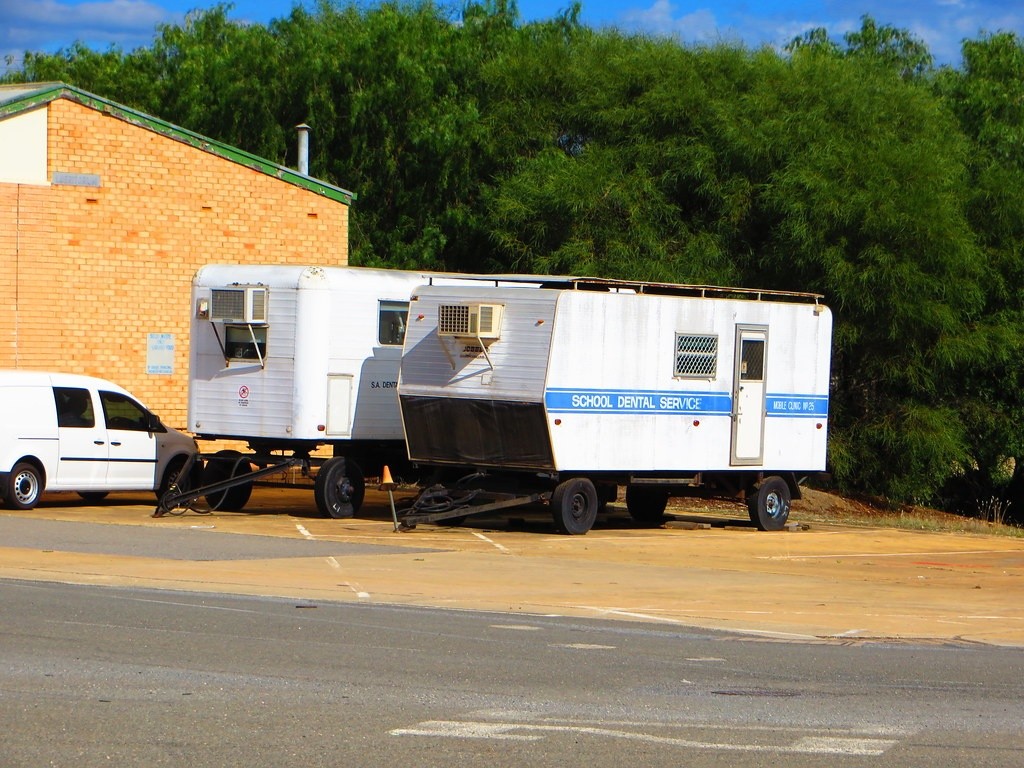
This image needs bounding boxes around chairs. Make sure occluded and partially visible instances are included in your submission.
[63,396,90,426]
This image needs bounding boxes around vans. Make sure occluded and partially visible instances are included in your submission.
[0,370,201,509]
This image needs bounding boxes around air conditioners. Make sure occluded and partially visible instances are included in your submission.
[209,283,269,323]
[437,303,504,341]
